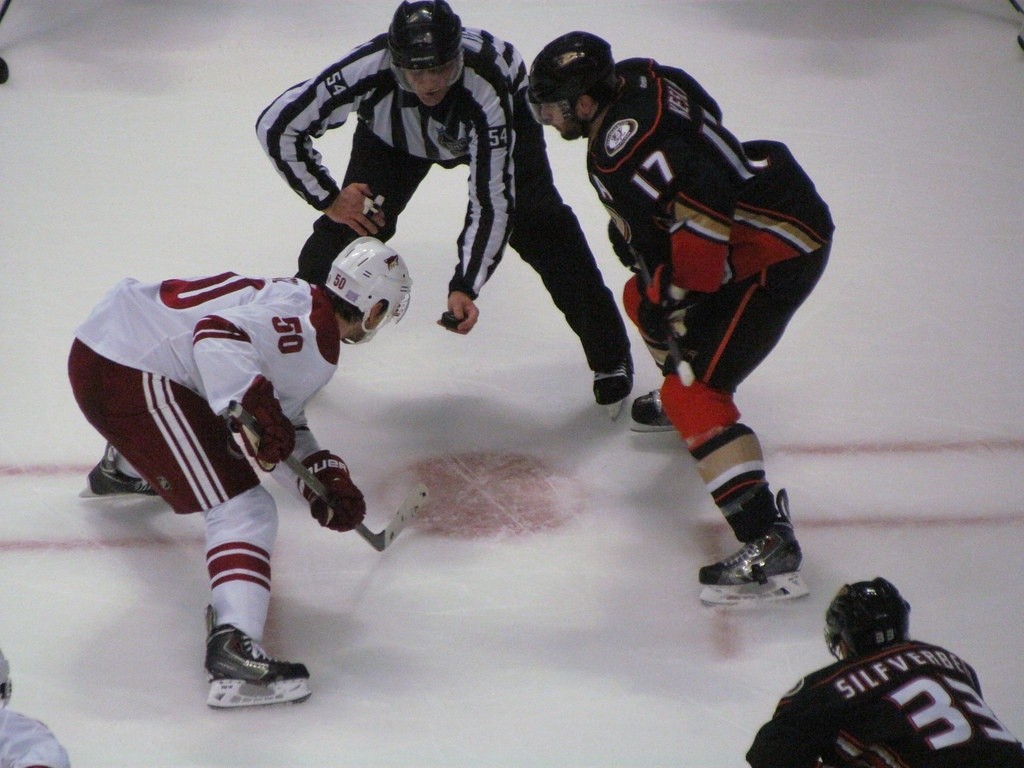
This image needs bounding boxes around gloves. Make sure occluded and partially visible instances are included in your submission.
[638,283,719,345]
[608,216,658,271]
[297,450,367,532]
[228,375,296,463]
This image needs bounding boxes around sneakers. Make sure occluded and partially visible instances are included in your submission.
[79,441,159,501]
[698,488,810,603]
[630,386,679,433]
[205,604,311,708]
[593,351,633,421]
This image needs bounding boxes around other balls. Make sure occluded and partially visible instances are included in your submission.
[441,308,471,328]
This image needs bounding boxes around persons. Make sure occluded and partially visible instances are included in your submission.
[253,0,635,425]
[0,646,70,768]
[747,574,1024,768]
[68,237,413,710]
[523,30,834,605]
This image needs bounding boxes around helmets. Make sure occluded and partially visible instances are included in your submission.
[527,31,616,110]
[325,236,412,331]
[824,577,911,660]
[0,650,12,709]
[388,0,463,72]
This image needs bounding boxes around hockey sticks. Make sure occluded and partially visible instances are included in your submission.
[227,399,430,550]
[619,241,697,388]
